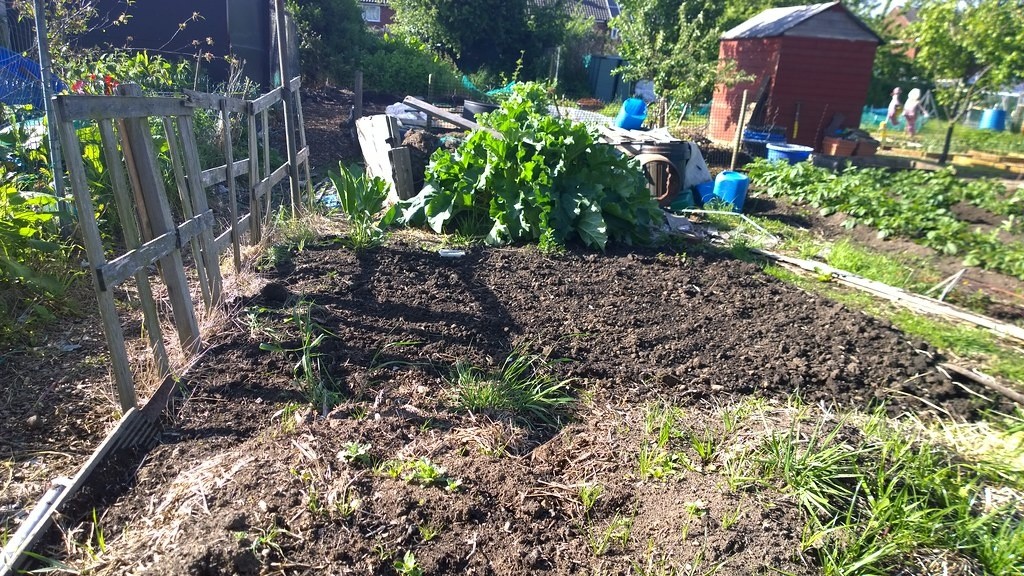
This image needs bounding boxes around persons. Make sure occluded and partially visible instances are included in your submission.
[904,88,928,136]
[886,87,903,125]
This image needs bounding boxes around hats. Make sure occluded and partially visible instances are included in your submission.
[890,87,903,96]
[908,87,921,101]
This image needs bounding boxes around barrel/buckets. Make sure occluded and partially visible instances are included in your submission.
[713,171,749,211]
[766,142,814,166]
[980,109,1006,131]
[618,97,647,130]
[670,190,694,210]
[695,180,715,207]
[460,99,501,133]
